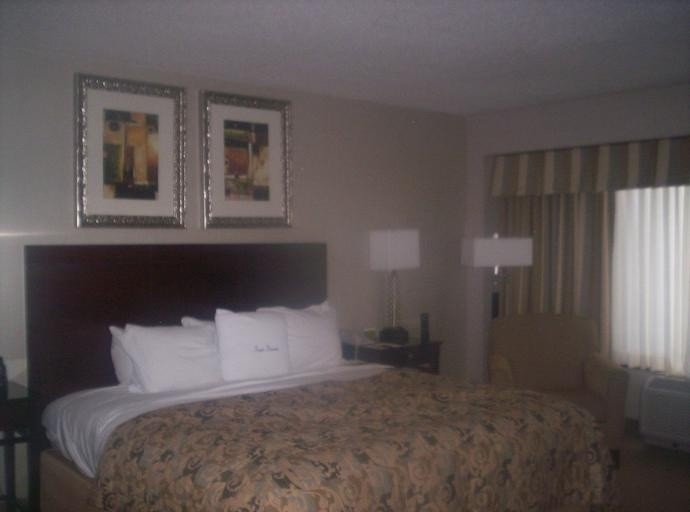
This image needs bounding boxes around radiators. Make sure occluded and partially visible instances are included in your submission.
[636,374,690,454]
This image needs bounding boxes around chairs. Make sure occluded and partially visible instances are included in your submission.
[485,311,631,468]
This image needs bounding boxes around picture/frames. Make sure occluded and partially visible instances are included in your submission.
[198,89,293,227]
[70,72,187,230]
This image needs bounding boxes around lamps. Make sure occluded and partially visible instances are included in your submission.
[368,226,420,342]
[472,230,533,316]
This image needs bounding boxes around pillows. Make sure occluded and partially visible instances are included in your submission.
[106,323,154,395]
[214,308,291,380]
[122,321,223,391]
[261,297,344,373]
[180,316,214,327]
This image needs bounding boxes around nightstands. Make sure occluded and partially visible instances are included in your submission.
[0,359,31,512]
[343,336,444,374]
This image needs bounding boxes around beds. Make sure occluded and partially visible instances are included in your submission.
[23,242,622,512]
